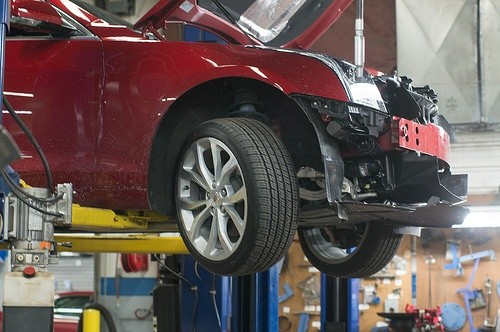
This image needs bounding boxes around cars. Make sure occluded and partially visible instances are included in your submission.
[1,0,469,277]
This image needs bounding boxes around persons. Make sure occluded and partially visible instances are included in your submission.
[372,291,379,304]
